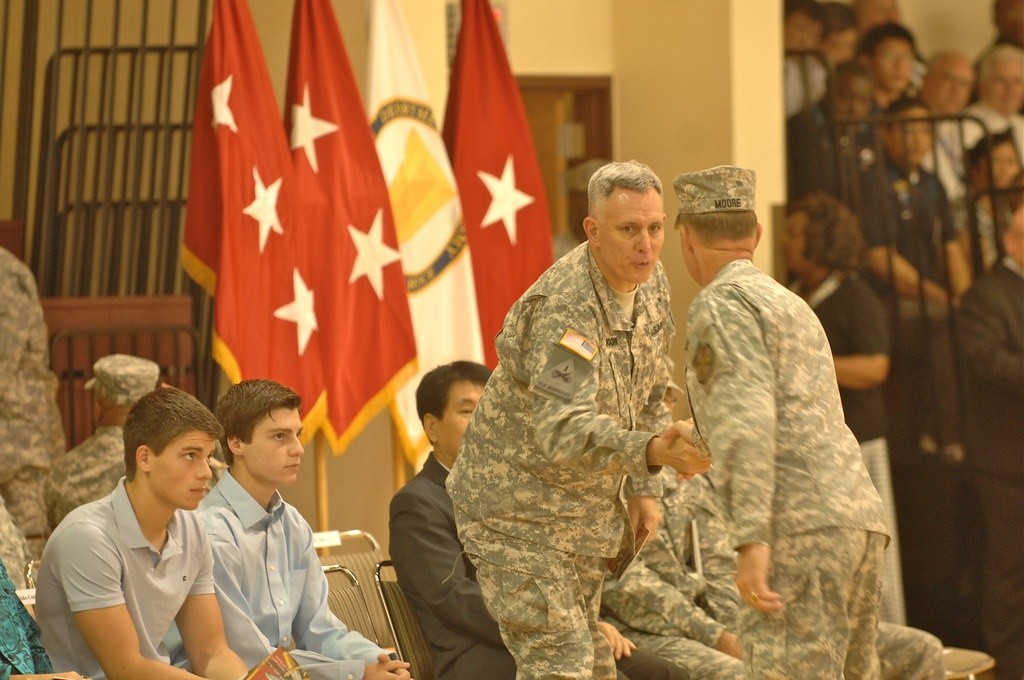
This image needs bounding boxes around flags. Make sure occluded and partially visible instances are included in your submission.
[181,0,553,465]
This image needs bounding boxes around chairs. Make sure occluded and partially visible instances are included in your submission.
[319,565,381,647]
[372,561,436,680]
[311,530,395,649]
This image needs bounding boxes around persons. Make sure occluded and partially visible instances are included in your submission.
[0,558,87,680]
[600,356,947,680]
[0,247,224,593]
[389,360,690,680]
[35,387,248,680]
[785,0,1024,680]
[660,165,893,680]
[446,160,710,680]
[162,380,411,680]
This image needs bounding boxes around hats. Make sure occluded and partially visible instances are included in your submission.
[673,164,761,217]
[85,352,161,408]
